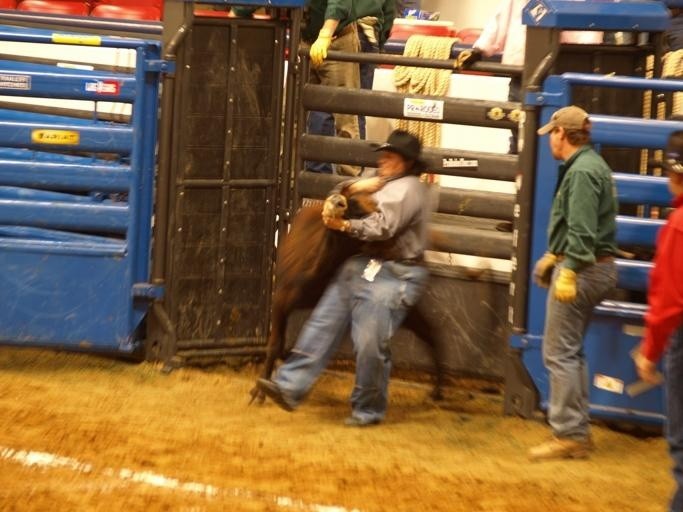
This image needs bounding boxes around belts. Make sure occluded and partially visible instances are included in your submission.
[556,254,613,264]
[332,22,357,41]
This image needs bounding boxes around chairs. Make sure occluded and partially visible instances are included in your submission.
[194,11,272,19]
[382,19,501,77]
[2,1,164,24]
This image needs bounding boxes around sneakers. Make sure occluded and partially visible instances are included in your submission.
[528,432,595,459]
[257,378,293,411]
[497,222,513,232]
[346,416,380,426]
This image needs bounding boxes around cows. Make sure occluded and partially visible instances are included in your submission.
[248,177,451,409]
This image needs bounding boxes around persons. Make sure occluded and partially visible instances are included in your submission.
[529,105,617,460]
[453,0,603,231]
[229,0,362,175]
[632,130,683,512]
[302,0,396,174]
[256,130,428,426]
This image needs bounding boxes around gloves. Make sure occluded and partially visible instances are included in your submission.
[554,266,577,304]
[534,253,556,288]
[454,48,480,70]
[309,30,332,65]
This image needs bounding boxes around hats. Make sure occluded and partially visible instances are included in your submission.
[376,129,420,171]
[537,105,591,135]
[646,131,683,174]
